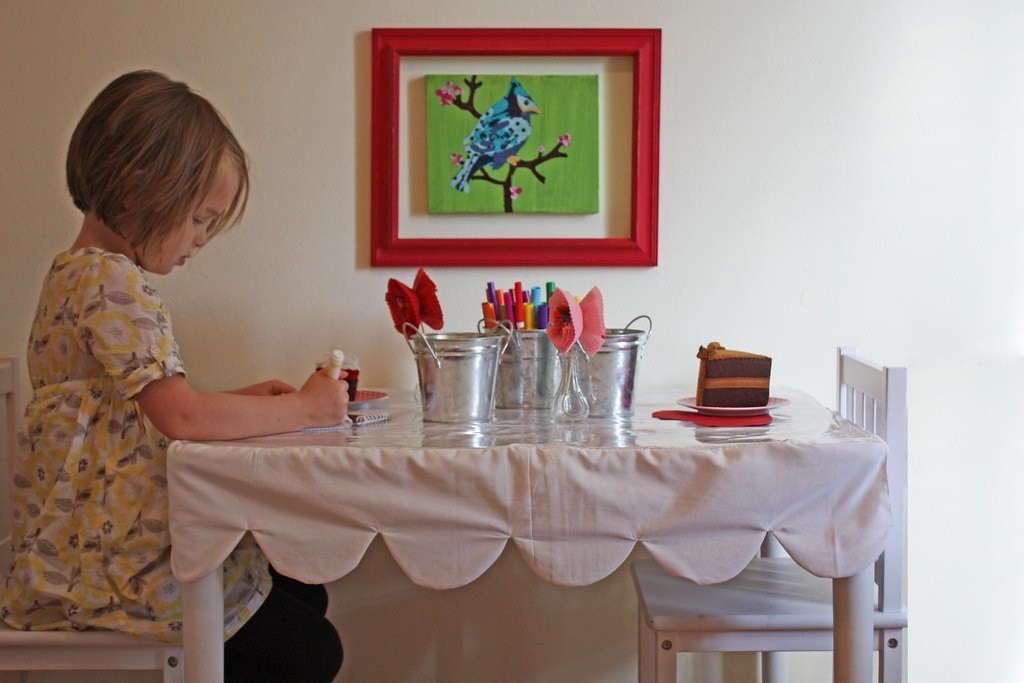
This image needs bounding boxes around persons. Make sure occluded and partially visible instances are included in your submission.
[0,71,350,683]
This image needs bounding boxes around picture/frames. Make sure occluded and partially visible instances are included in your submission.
[369,26,662,269]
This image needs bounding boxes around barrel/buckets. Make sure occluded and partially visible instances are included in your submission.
[483,321,567,410]
[400,320,512,423]
[574,315,652,417]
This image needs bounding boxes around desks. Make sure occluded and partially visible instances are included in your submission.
[166,388,894,683]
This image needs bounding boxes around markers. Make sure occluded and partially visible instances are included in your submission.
[481,281,554,331]
[328,348,345,382]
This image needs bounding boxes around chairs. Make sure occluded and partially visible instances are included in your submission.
[628,345,909,683]
[0,356,185,683]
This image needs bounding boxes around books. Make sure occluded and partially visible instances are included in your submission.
[302,412,390,431]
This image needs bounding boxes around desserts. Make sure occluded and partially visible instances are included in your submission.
[315,352,360,402]
[696,341,772,409]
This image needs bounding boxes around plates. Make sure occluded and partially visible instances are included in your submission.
[675,396,790,415]
[348,389,390,412]
